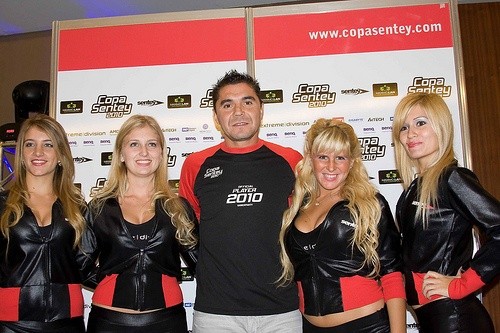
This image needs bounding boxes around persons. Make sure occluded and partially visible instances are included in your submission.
[79,115,200,332]
[179,71,306,332]
[391,90,500,333]
[0,115,94,332]
[281,117,407,333]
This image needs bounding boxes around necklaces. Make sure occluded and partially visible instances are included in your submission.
[315,197,323,206]
